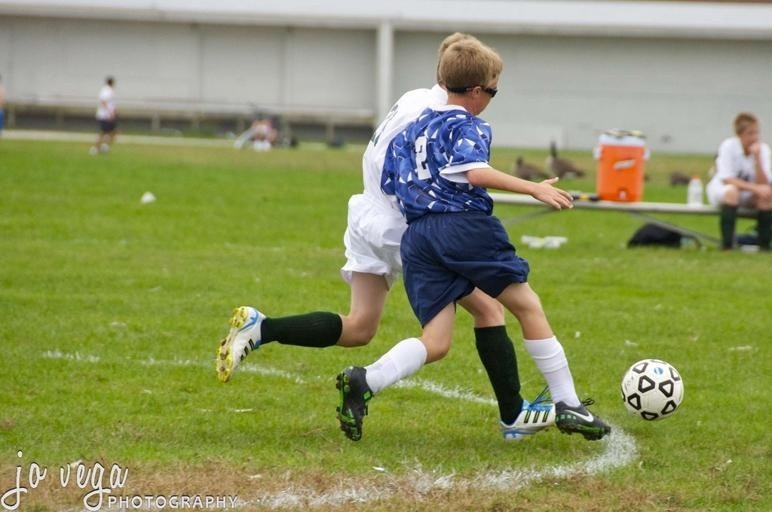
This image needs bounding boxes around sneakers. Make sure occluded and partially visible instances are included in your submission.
[335,366,372,441]
[216,306,267,382]
[556,397,611,440]
[499,387,555,440]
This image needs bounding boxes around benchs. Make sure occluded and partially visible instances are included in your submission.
[487,192,759,251]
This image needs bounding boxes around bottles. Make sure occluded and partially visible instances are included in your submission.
[687,175,704,209]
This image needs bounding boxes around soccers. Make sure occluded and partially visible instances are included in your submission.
[621,359,683,422]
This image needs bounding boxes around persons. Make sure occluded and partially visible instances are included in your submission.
[251,106,273,151]
[545,142,586,180]
[335,37,612,448]
[707,114,771,250]
[95,76,121,155]
[217,31,555,441]
[515,157,551,181]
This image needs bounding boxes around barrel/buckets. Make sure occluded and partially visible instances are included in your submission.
[595,134,651,201]
[686,175,703,204]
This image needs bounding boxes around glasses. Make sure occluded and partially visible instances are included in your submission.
[448,82,498,99]
